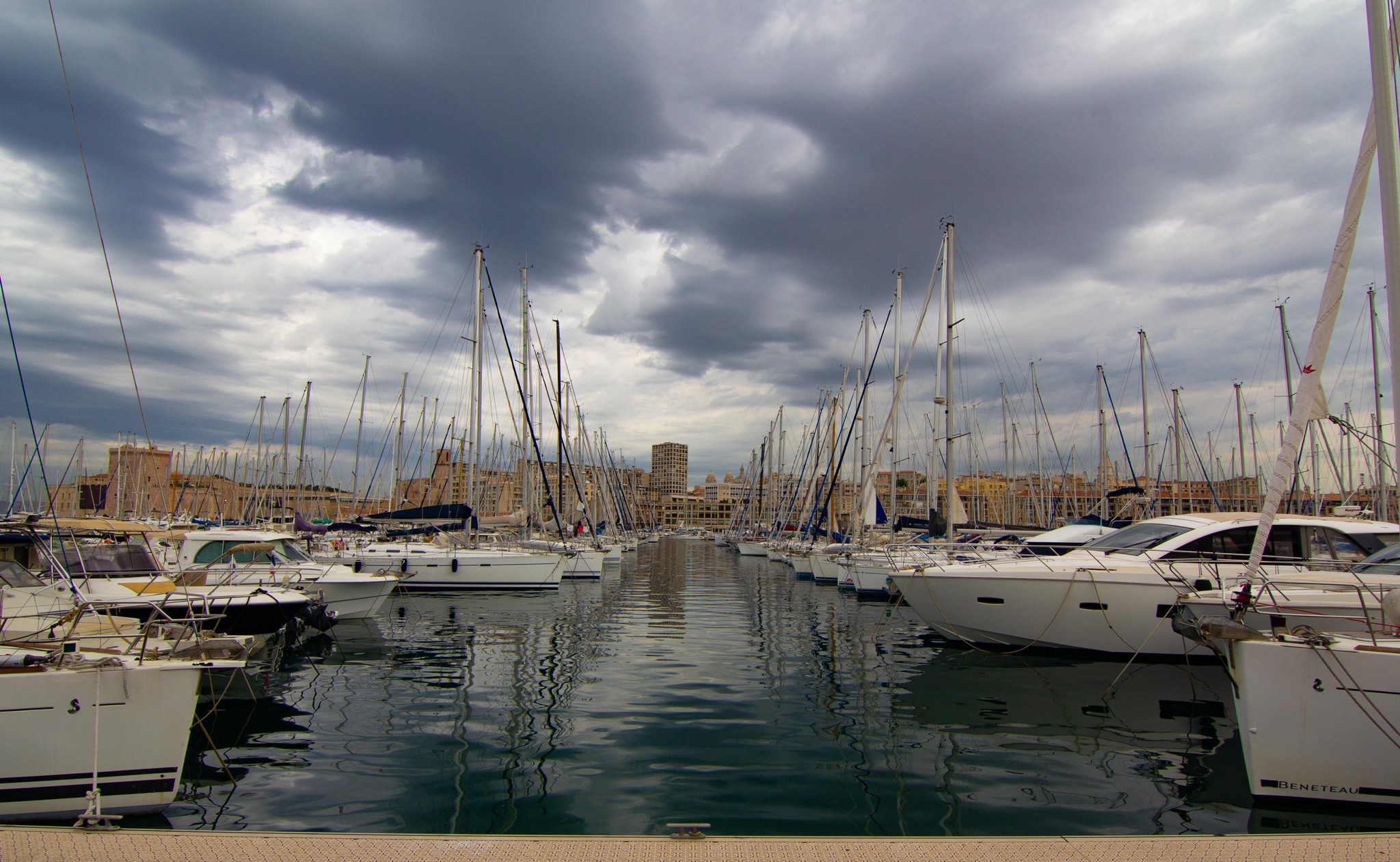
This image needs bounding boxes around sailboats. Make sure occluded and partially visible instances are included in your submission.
[711,0,1399,811]
[0,1,705,824]
